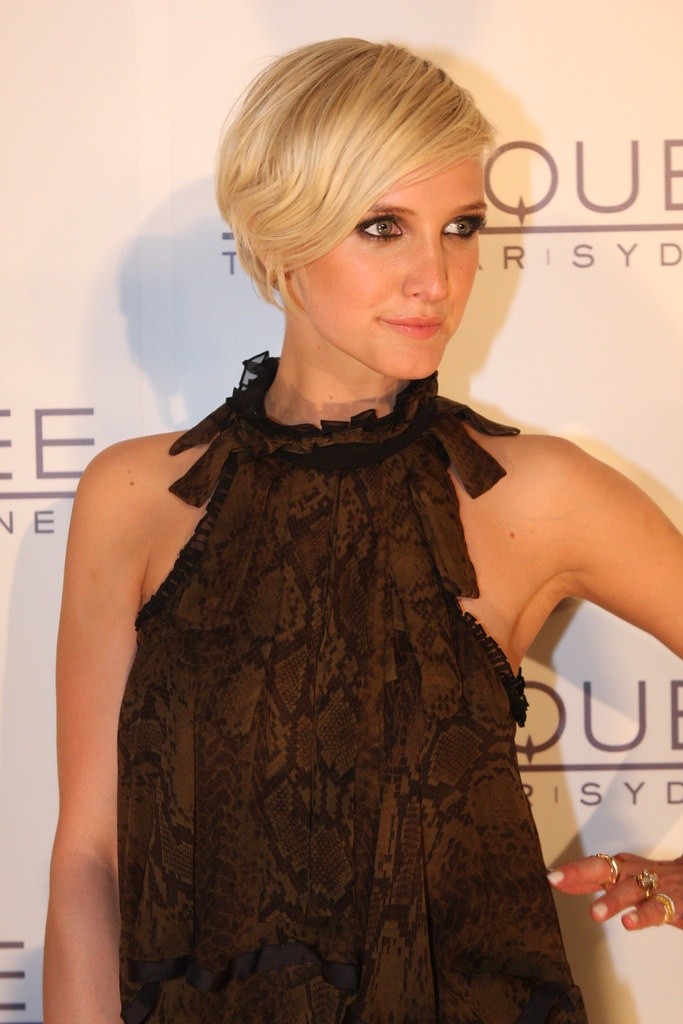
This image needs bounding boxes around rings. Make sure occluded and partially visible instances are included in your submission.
[596,853,618,886]
[655,894,674,926]
[630,869,658,898]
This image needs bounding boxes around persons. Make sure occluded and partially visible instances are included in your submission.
[44,37,683,1024]
